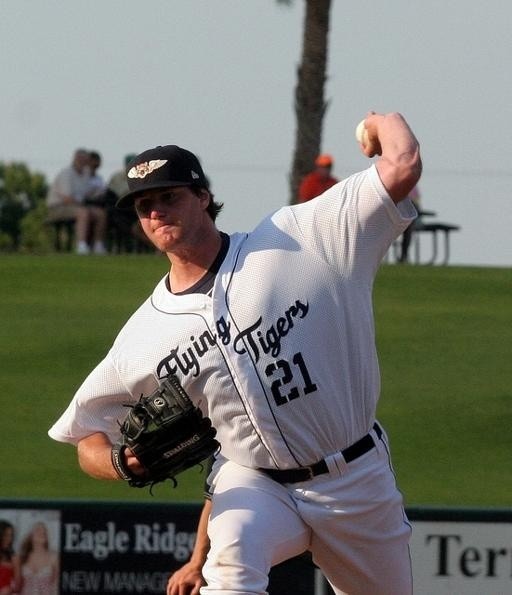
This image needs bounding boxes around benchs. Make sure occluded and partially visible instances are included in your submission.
[385,213,460,265]
[54,216,158,253]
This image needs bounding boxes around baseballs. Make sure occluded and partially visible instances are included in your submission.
[356,119,369,147]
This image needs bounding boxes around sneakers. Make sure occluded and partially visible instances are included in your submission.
[74,240,107,256]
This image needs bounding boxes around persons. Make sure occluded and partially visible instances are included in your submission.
[167,449,227,595]
[298,154,337,204]
[42,149,137,255]
[2,521,20,594]
[47,113,425,595]
[21,520,57,595]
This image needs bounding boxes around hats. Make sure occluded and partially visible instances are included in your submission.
[115,146,209,203]
[315,155,332,168]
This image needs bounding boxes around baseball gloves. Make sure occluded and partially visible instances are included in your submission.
[111,375,220,489]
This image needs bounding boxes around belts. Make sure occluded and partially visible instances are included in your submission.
[258,422,383,484]
